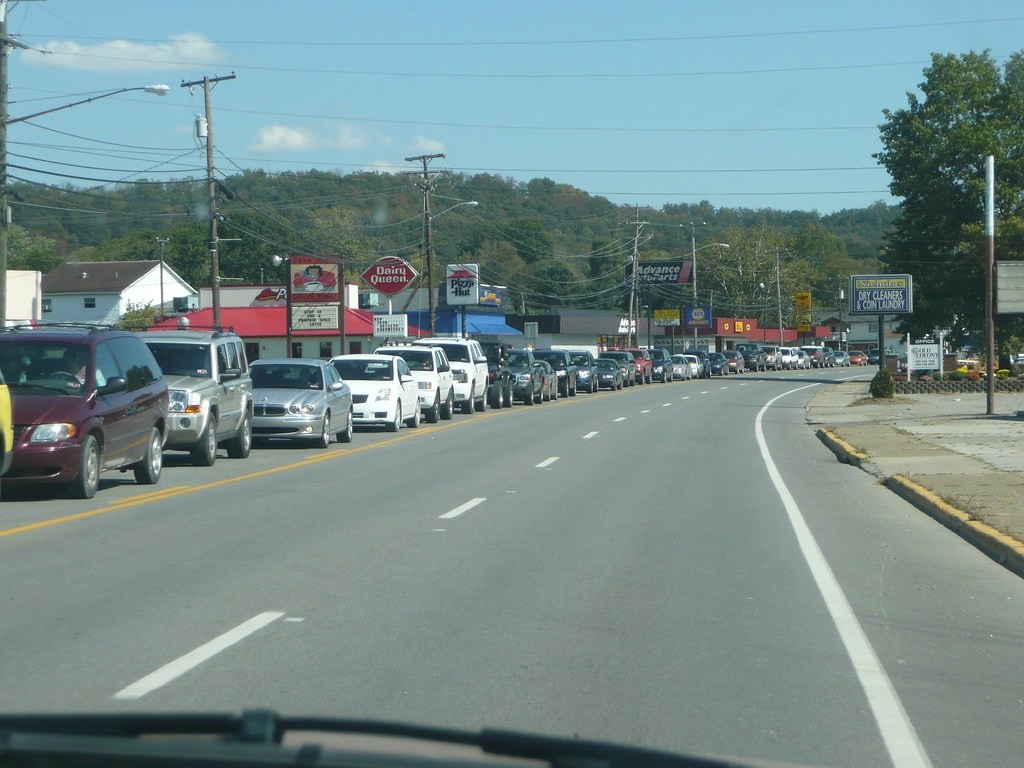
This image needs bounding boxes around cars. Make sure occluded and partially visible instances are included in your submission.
[247,359,354,449]
[507,344,881,406]
[328,354,422,432]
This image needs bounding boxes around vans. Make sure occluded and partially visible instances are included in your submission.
[411,337,492,415]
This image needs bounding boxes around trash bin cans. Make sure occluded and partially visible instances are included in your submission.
[885,354,898,371]
[943,353,958,371]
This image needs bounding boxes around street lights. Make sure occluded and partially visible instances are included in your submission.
[424,200,479,337]
[692,242,731,349]
[0,83,172,319]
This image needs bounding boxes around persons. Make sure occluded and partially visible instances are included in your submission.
[516,355,528,368]
[485,347,496,356]
[41,346,107,388]
[413,353,432,369]
[554,356,564,366]
[605,363,610,369]
[621,357,628,364]
[635,353,642,359]
[655,353,663,361]
[292,369,320,389]
[579,357,587,365]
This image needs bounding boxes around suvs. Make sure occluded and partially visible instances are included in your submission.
[0,321,170,500]
[374,340,456,424]
[470,337,515,410]
[118,324,255,468]
